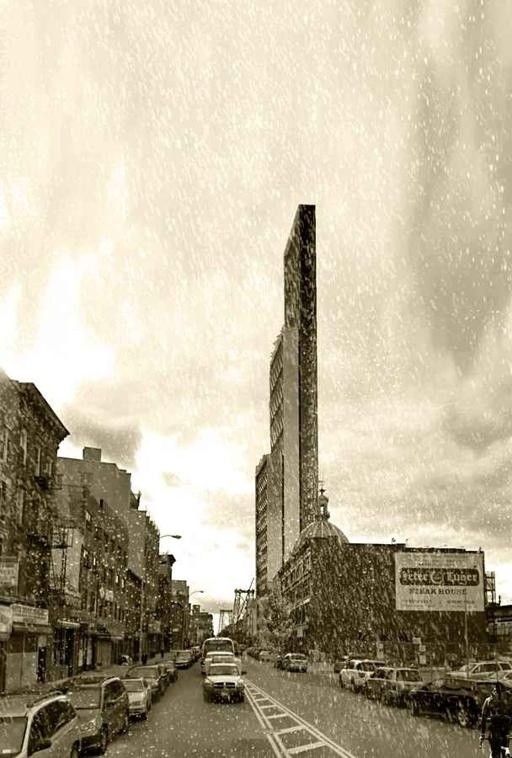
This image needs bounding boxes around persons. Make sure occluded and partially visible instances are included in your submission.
[481,683,512,758]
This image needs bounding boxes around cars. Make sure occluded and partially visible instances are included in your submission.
[173,646,201,668]
[334,656,349,672]
[281,653,308,671]
[247,647,273,660]
[119,661,177,719]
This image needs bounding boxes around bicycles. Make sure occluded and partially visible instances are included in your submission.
[479,737,512,758]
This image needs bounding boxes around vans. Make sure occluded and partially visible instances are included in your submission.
[201,638,247,700]
[404,678,506,728]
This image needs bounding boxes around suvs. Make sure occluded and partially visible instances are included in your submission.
[444,661,512,688]
[57,677,130,754]
[1,690,82,758]
[364,667,423,707]
[338,660,386,693]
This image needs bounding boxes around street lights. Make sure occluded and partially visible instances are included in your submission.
[181,590,204,650]
[139,534,180,664]
